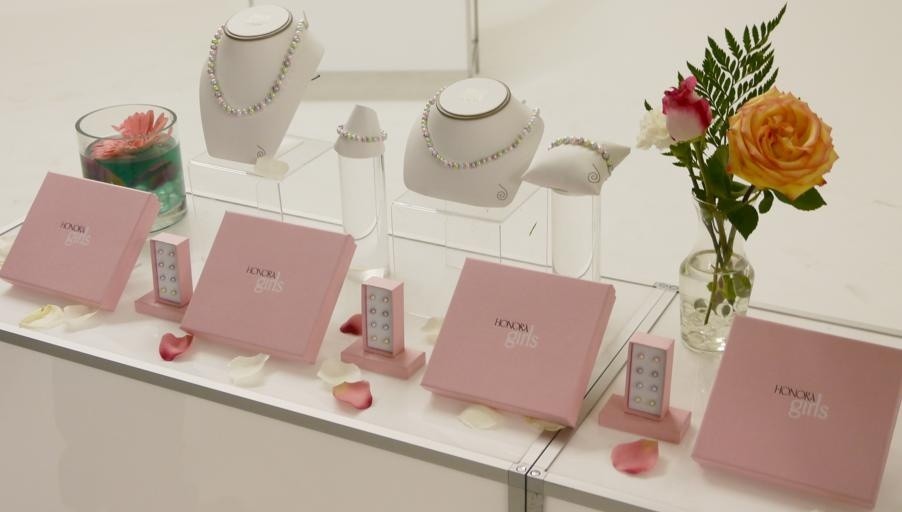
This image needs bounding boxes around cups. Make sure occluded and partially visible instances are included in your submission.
[73,104,189,235]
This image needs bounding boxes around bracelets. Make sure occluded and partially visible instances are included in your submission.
[335,126,388,143]
[546,138,614,173]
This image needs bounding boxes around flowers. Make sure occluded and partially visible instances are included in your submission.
[84,111,176,163]
[643,4,841,318]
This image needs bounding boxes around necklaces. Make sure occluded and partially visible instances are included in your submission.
[421,84,540,168]
[206,19,306,116]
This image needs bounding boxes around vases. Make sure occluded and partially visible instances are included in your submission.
[677,248,756,356]
[74,104,188,235]
